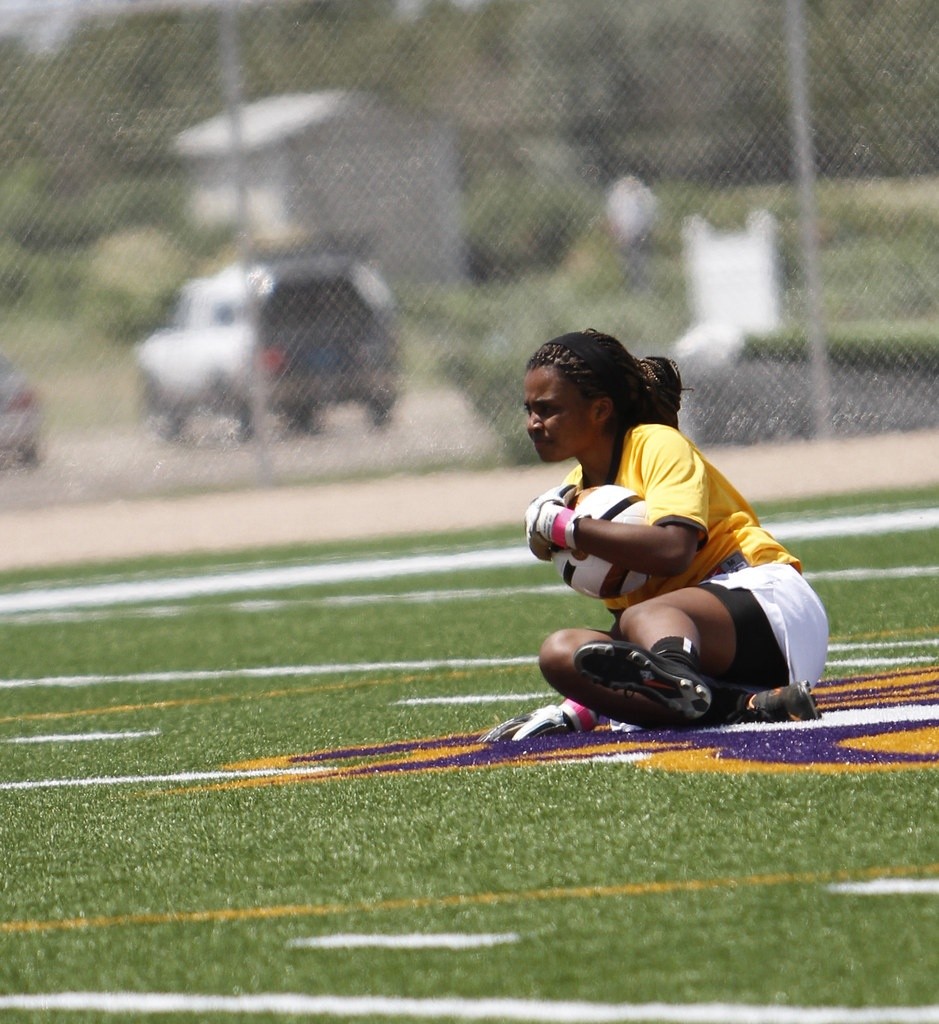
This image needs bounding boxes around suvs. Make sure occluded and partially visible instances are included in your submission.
[135,254,406,445]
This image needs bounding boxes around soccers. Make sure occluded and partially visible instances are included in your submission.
[548,482,655,600]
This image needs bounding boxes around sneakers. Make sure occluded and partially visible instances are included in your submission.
[725,679,823,725]
[575,641,710,719]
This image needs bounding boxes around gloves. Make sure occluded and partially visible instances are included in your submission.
[476,703,584,743]
[524,483,578,562]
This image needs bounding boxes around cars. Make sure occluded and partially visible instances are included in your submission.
[0,346,55,471]
[651,312,825,460]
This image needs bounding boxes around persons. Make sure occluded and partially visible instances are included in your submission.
[476,330,828,743]
